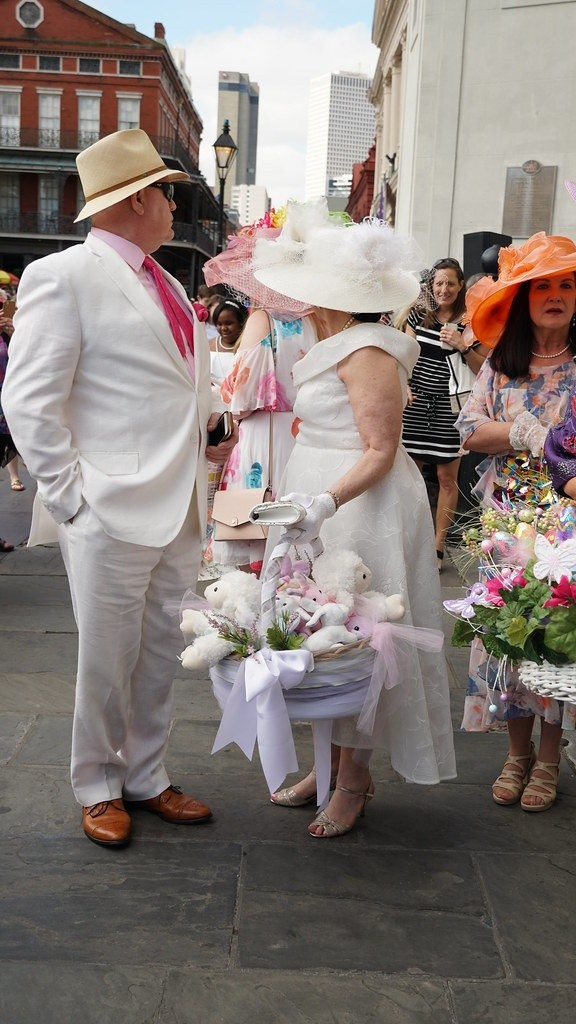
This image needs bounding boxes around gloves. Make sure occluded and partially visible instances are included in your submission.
[280,492,336,543]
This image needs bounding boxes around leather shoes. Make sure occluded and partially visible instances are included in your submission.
[82,798,131,844]
[127,784,212,824]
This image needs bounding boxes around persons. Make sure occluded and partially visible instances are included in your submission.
[0,287,32,491]
[201,218,331,580]
[452,231,576,811]
[0,537,15,552]
[542,390,576,501]
[381,243,501,575]
[0,128,238,848]
[195,283,250,580]
[250,198,456,838]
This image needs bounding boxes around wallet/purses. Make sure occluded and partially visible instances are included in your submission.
[247,501,304,526]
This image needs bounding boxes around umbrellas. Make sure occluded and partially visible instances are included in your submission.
[0,270,20,284]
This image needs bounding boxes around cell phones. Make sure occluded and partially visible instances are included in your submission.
[3,300,15,318]
[210,411,234,447]
[441,322,458,350]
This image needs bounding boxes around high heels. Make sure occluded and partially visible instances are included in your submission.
[491,741,537,803]
[309,775,375,837]
[520,752,561,811]
[270,768,337,806]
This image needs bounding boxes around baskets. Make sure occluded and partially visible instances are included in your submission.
[208,529,389,720]
[517,649,576,703]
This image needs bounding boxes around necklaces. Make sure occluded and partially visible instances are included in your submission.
[531,342,570,358]
[219,335,235,351]
[341,316,356,330]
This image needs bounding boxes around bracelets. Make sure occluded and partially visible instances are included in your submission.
[462,345,471,356]
[325,490,340,511]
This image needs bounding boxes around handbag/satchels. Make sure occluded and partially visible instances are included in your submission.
[212,490,274,541]
[445,352,477,414]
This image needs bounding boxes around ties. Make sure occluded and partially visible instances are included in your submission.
[141,256,194,361]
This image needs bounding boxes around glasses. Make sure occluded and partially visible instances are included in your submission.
[432,258,460,268]
[147,181,174,202]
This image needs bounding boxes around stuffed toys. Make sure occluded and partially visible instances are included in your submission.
[176,547,405,671]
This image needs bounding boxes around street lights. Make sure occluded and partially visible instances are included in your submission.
[212,118,239,298]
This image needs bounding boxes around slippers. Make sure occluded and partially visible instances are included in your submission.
[10,480,24,490]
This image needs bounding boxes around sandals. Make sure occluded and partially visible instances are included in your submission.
[0,537,16,552]
[436,548,444,573]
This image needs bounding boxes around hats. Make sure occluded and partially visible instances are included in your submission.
[73,129,189,223]
[252,195,448,314]
[461,231,576,348]
[481,243,503,276]
[203,206,315,322]
